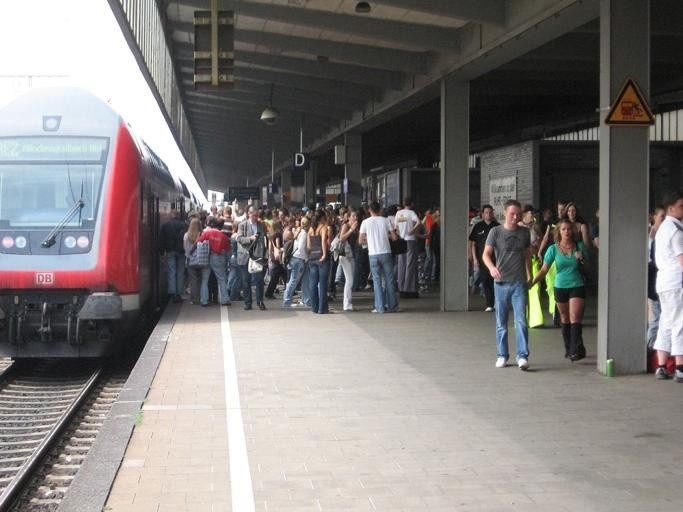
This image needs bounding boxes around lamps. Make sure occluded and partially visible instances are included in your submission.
[255,84,278,126]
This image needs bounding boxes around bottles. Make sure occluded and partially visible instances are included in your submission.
[607,359,615,377]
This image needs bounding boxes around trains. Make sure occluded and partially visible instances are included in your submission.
[0,75,202,371]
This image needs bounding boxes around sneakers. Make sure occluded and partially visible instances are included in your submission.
[171,280,434,313]
[673,368,683,382]
[485,306,494,312]
[518,358,530,370]
[655,366,668,380]
[495,356,507,368]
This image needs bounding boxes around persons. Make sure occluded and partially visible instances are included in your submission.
[481,200,534,369]
[526,218,593,363]
[652,190,683,384]
[646,206,665,362]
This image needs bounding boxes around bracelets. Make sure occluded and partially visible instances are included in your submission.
[579,256,585,265]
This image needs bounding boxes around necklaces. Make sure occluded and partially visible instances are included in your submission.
[559,240,574,249]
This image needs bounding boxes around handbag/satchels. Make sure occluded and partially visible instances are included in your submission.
[247,258,264,274]
[577,243,593,283]
[390,235,408,255]
[329,233,346,259]
[272,240,282,262]
[249,232,268,265]
[188,239,210,268]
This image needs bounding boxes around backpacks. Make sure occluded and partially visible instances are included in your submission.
[282,234,296,268]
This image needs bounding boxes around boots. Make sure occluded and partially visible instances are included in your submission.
[561,323,572,357]
[570,322,586,361]
